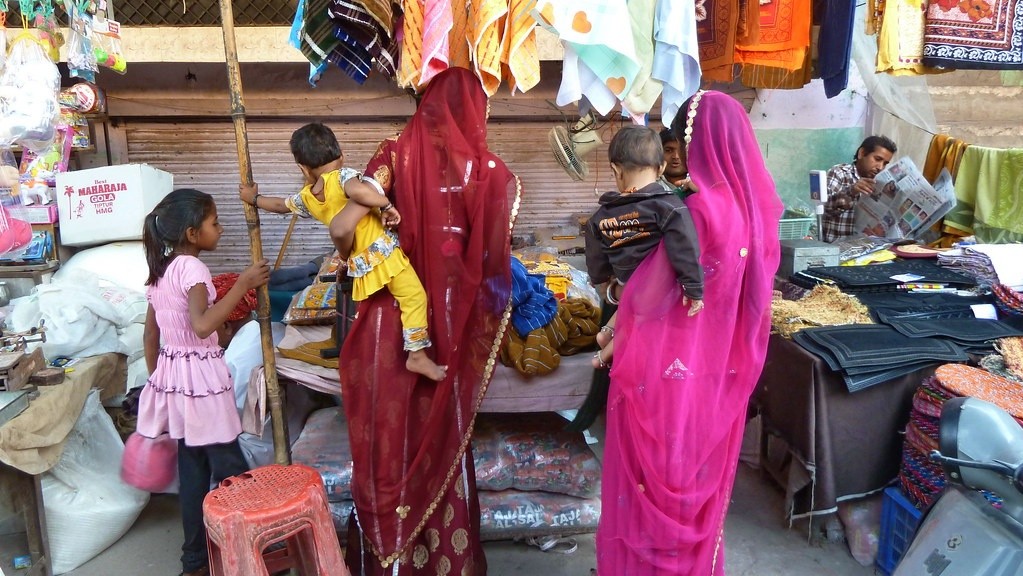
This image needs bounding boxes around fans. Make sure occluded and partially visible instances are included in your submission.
[547,113,603,182]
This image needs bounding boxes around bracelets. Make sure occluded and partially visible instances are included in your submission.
[252,194,260,208]
[380,202,393,212]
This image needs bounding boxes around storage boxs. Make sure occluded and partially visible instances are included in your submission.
[876,487,925,576]
[0,125,174,265]
[776,239,840,280]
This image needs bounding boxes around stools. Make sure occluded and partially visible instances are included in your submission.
[203,464,351,576]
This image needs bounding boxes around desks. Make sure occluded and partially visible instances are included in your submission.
[761,321,987,540]
[0,352,129,576]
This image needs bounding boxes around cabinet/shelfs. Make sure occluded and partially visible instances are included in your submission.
[0,85,112,287]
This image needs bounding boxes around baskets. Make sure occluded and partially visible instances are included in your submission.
[873,486,925,575]
[779,209,817,240]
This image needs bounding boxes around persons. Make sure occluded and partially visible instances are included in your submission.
[581,123,706,362]
[596,87,783,576]
[337,67,524,576]
[238,122,448,383]
[136,188,271,576]
[658,126,688,191]
[822,135,897,246]
